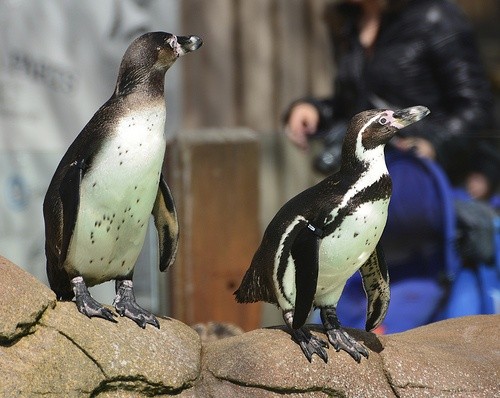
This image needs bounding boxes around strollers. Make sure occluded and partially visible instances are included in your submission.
[310,151,499,336]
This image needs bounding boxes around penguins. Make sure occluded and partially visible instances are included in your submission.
[230,102,432,363]
[41,31,204,331]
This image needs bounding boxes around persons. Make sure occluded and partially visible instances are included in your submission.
[281,0,487,164]
